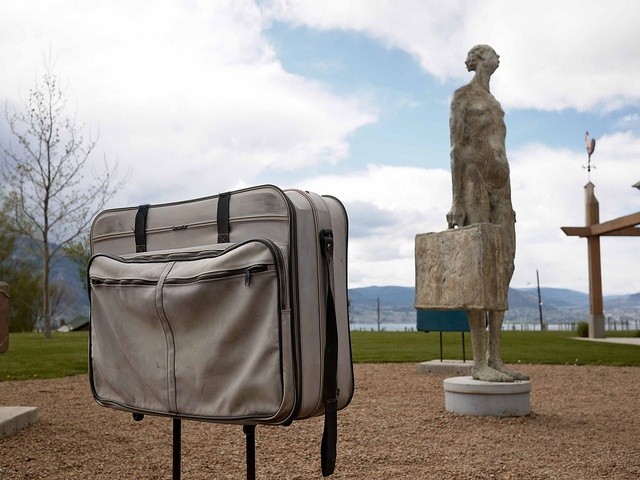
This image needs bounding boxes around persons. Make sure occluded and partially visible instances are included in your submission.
[414,44,532,382]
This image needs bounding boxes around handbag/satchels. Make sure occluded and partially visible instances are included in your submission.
[86,184,356,480]
